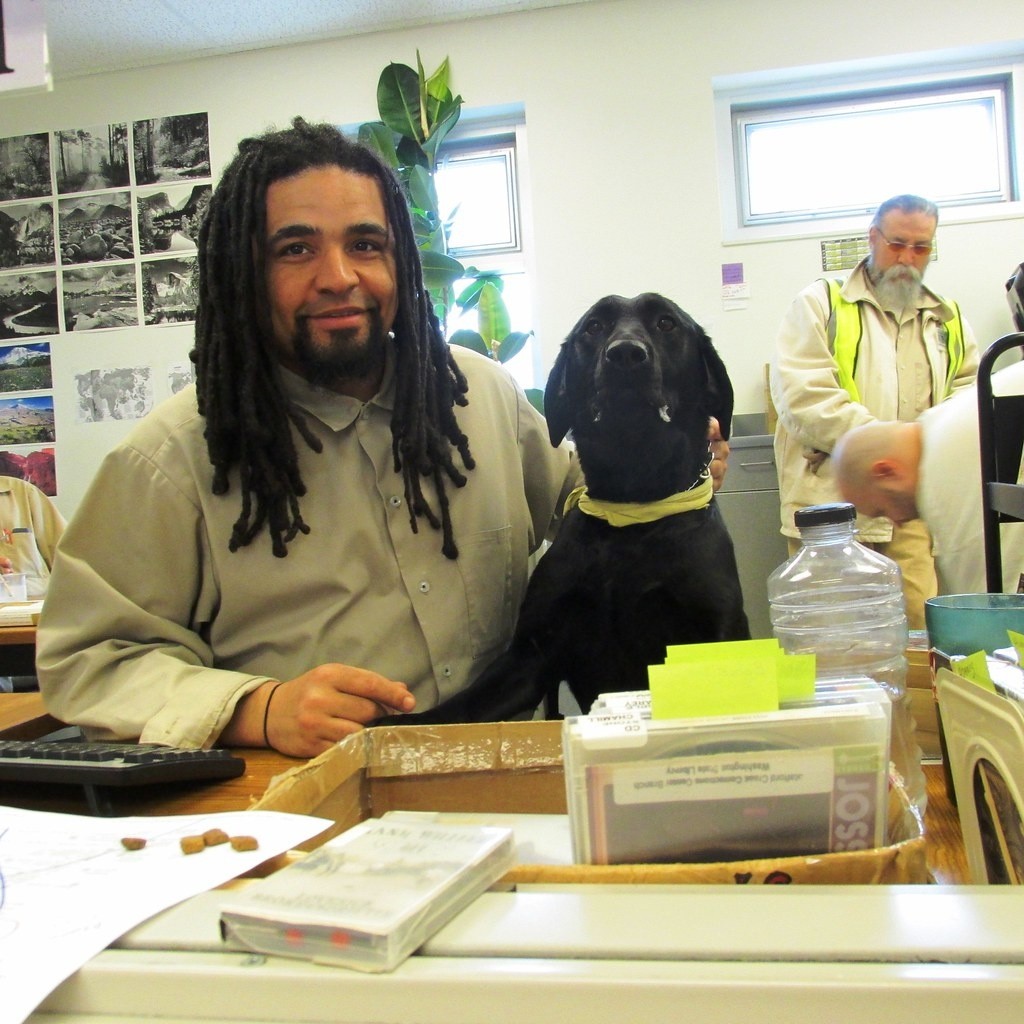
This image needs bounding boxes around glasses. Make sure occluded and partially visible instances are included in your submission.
[877,228,932,257]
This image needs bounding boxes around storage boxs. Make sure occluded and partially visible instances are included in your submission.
[245,724,930,887]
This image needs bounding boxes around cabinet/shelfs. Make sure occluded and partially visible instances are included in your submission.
[714,446,790,640]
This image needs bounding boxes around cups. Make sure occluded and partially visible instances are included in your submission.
[926,593,1022,805]
[0,572,28,602]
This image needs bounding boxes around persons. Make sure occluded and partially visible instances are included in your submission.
[0,476,67,693]
[770,196,981,629]
[35,117,730,757]
[831,360,1024,596]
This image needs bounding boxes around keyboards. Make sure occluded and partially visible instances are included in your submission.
[0,741,246,814]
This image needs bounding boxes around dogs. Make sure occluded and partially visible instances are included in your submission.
[364,290,753,729]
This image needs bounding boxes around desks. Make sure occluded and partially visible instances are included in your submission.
[0,690,972,886]
[0,601,38,646]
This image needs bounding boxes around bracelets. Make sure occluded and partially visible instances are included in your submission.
[263,682,284,748]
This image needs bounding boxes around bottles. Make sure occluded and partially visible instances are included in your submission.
[767,504,907,700]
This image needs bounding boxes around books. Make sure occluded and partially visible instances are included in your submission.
[222,818,518,971]
